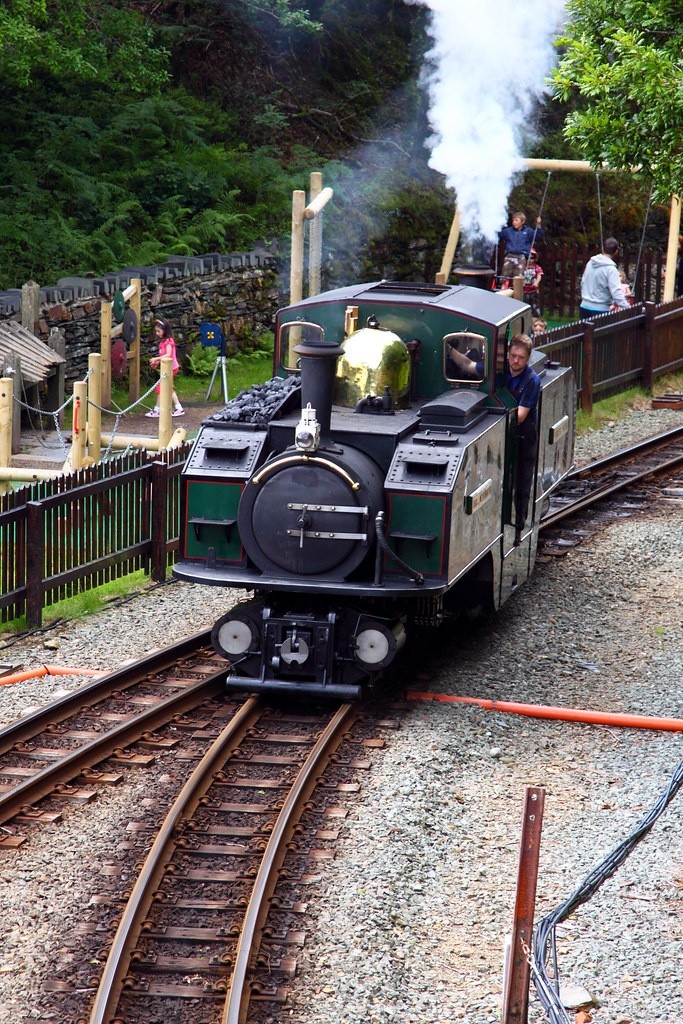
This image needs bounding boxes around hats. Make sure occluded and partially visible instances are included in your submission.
[531,248,537,261]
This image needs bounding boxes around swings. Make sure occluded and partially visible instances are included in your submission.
[491,170,553,292]
[595,171,655,312]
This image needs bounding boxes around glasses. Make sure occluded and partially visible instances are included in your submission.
[530,257,533,259]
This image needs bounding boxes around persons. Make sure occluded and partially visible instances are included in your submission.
[145,318,185,417]
[498,212,548,335]
[580,239,634,319]
[446,336,540,547]
[662,235,683,297]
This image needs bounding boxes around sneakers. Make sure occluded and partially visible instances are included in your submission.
[145,409,159,417]
[170,409,185,417]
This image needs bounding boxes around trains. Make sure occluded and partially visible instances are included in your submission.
[173,265,576,701]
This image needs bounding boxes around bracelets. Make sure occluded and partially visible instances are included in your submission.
[447,347,453,356]
